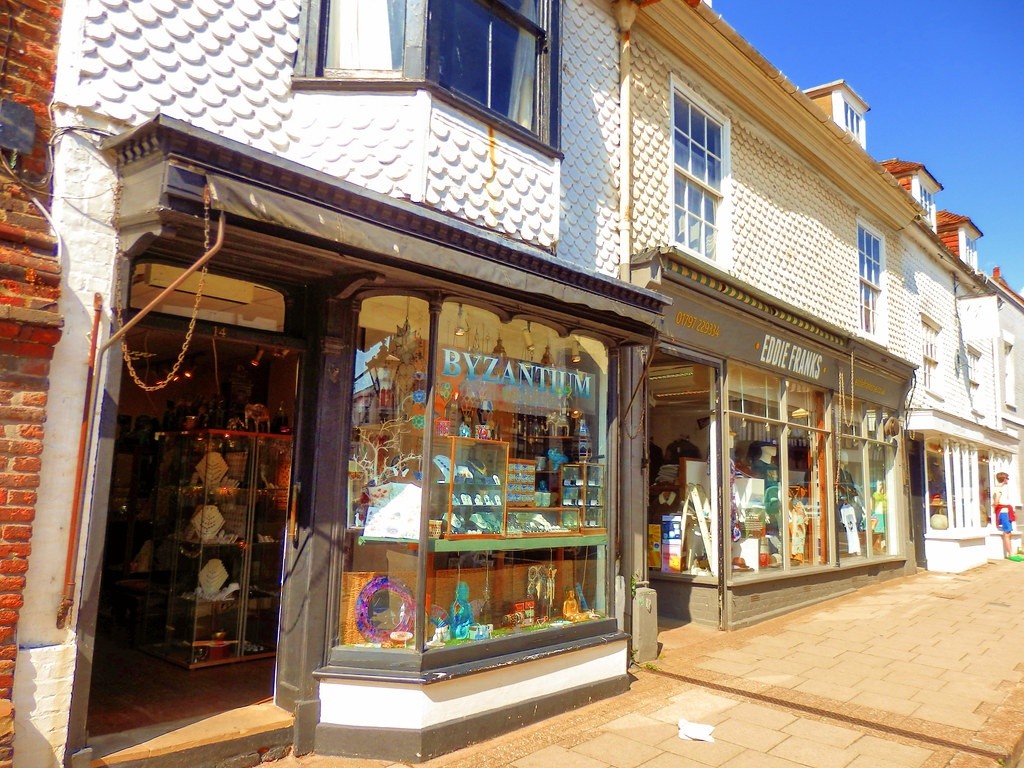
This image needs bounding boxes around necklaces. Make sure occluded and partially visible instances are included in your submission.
[434,455,555,532]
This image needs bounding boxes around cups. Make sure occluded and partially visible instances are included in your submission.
[535,491,551,507]
[428,519,442,539]
[534,456,546,471]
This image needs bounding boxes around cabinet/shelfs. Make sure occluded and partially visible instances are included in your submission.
[428,437,606,541]
[100,431,294,670]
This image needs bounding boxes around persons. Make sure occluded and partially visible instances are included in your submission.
[871,480,885,555]
[991,472,1024,562]
[563,587,589,621]
[751,437,778,553]
[838,449,855,499]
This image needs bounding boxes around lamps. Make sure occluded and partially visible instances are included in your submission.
[151,371,179,384]
[739,369,747,428]
[273,349,290,358]
[455,304,465,336]
[764,374,769,431]
[571,342,581,362]
[185,366,197,377]
[523,320,535,351]
[251,350,265,365]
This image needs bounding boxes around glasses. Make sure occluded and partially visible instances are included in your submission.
[1006,478,1009,480]
